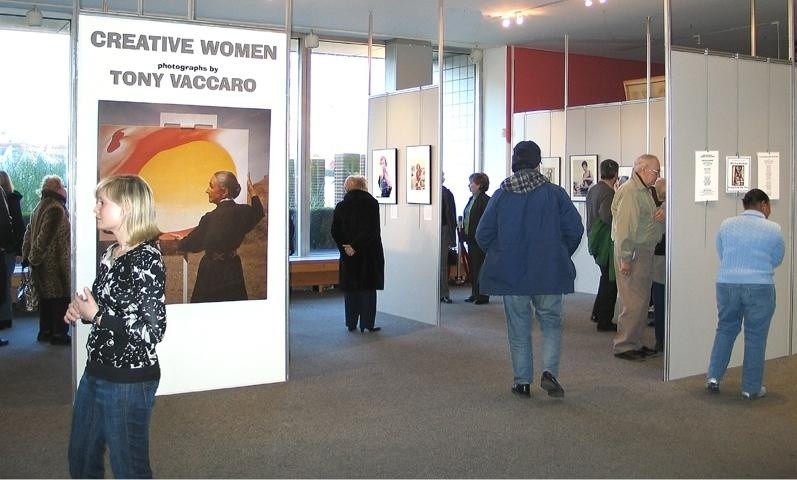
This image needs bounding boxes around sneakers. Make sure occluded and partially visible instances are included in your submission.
[50,334,71,344]
[704,378,720,392]
[740,386,766,401]
[38,331,49,341]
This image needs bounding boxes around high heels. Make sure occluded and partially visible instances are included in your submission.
[360,327,380,332]
[348,325,355,331]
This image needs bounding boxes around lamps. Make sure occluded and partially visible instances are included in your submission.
[26,4,42,26]
[470,44,483,62]
[305,29,319,49]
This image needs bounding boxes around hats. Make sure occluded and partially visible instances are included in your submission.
[512,141,540,171]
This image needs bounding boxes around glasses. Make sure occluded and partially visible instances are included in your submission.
[646,169,660,177]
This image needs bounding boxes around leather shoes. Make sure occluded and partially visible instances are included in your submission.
[473,299,489,303]
[465,296,476,302]
[443,296,452,303]
[598,324,616,331]
[541,371,564,397]
[511,383,530,399]
[614,351,644,362]
[639,347,656,357]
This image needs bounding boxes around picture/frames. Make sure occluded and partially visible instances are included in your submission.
[405,145,431,205]
[371,148,397,205]
[538,157,561,187]
[618,167,633,187]
[568,154,599,202]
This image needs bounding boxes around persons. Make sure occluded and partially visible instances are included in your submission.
[376,157,392,198]
[579,160,595,186]
[169,169,265,303]
[475,141,585,401]
[440,170,458,304]
[413,163,425,191]
[586,153,666,362]
[0,169,25,332]
[699,186,787,400]
[60,174,167,480]
[462,172,492,305]
[329,172,386,334]
[20,174,72,346]
[0,185,11,348]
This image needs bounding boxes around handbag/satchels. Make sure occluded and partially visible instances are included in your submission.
[448,248,457,265]
[16,277,40,313]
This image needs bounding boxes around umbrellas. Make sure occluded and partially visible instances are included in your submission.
[454,227,471,275]
